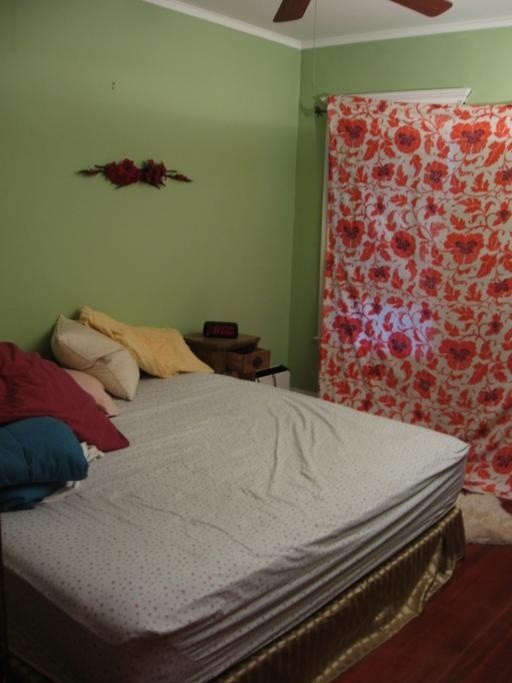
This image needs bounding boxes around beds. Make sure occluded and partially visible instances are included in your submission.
[0,373,469,683]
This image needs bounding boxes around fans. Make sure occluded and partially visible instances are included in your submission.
[273,0,452,22]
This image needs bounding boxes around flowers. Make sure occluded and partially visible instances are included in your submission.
[75,158,195,190]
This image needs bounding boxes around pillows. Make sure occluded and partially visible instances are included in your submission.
[1,339,130,512]
[49,312,142,402]
[78,306,213,377]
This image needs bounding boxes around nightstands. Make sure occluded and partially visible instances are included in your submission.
[184,333,270,375]
